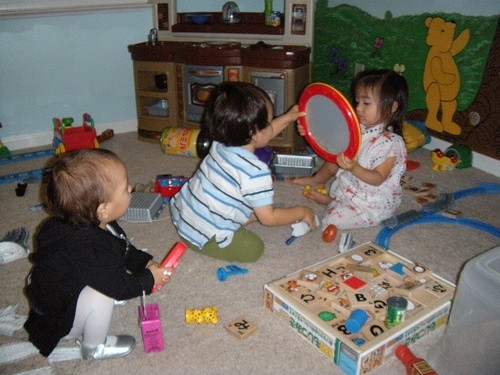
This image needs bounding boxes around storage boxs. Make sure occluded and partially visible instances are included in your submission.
[426,245,500,375]
[263,241,457,375]
[59,126,96,152]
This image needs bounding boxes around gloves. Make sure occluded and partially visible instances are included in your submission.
[0,227,31,265]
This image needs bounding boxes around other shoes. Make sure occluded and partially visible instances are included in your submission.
[80,334,136,360]
[114,299,128,306]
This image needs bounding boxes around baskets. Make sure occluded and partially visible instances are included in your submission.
[119,191,166,222]
[269,153,316,176]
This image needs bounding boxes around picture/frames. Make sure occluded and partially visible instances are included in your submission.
[289,3,306,35]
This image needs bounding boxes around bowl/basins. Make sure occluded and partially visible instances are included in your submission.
[187,14,212,25]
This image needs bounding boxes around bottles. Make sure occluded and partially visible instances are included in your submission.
[160,127,213,160]
[148,28,158,46]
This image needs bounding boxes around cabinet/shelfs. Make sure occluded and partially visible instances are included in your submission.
[128,39,312,153]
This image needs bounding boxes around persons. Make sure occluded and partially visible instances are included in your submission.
[168,81,316,263]
[24,147,176,362]
[286,68,409,232]
